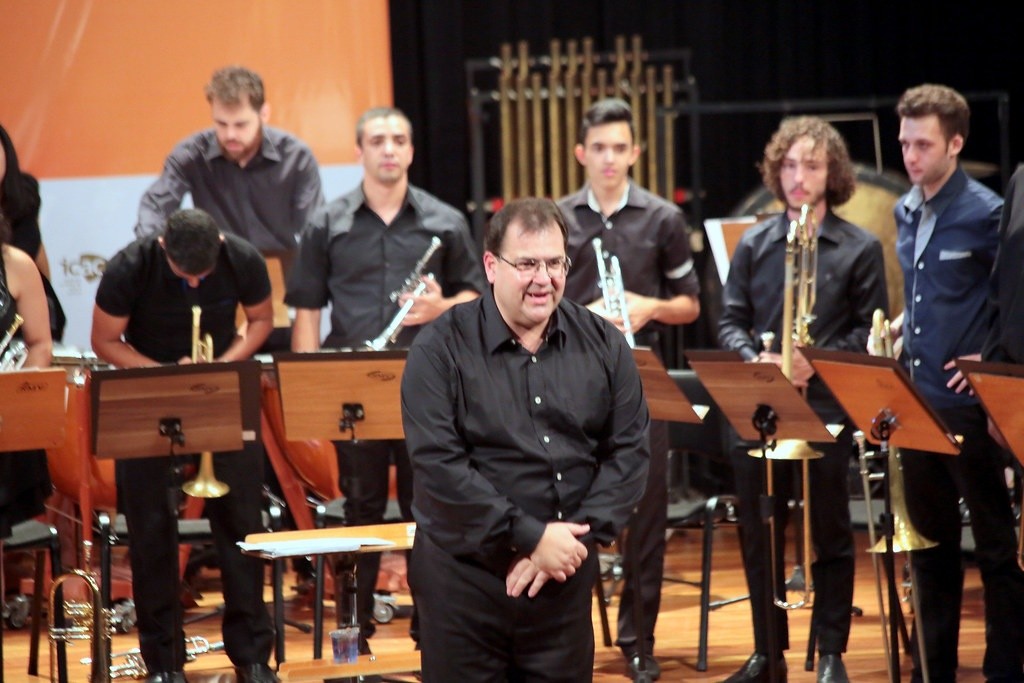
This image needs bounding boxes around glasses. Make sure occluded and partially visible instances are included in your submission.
[494,251,572,276]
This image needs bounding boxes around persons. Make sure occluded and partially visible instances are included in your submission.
[399,197,650,683]
[90,208,282,683]
[283,104,485,656]
[552,100,701,682]
[720,114,888,683]
[136,66,320,335]
[896,83,1024,683]
[0,125,66,541]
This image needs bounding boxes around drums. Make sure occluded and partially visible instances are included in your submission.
[254,348,400,502]
[50,350,201,515]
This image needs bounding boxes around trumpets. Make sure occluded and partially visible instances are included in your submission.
[0,313,30,370]
[46,539,116,683]
[181,304,231,499]
[592,236,636,349]
[363,236,443,349]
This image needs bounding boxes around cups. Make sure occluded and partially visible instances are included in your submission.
[329,628,361,665]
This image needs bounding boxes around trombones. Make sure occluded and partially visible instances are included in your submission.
[746,202,824,611]
[851,308,939,683]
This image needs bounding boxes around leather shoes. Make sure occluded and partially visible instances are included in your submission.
[627,650,661,683]
[816,653,849,683]
[721,649,788,683]
[144,667,190,683]
[237,662,281,683]
